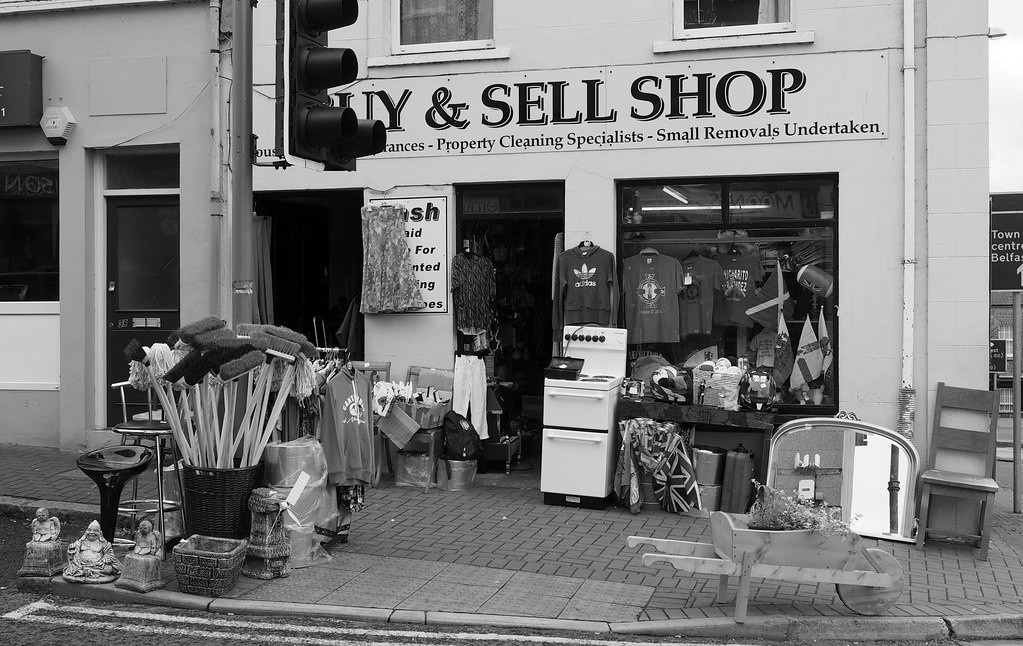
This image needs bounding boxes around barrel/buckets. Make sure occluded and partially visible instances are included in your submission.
[437,459,477,491]
[692,444,729,486]
[699,485,722,511]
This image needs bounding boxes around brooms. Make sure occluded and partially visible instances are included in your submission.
[123,340,183,464]
[160,315,318,475]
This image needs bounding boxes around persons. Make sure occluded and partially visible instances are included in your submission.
[66,520,117,575]
[30,508,57,542]
[135,520,157,555]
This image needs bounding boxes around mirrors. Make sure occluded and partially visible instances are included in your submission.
[766,411,921,544]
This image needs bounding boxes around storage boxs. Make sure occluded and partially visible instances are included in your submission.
[375,399,451,449]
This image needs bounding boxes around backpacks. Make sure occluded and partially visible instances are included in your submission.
[440,411,484,480]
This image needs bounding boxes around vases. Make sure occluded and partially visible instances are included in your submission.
[173,534,248,596]
[747,524,785,532]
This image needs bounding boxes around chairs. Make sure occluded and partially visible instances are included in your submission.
[351,359,457,495]
[916,381,1000,561]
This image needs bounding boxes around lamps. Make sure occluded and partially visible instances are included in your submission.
[661,186,689,204]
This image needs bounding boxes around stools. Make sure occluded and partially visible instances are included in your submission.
[76,444,154,545]
[110,379,186,560]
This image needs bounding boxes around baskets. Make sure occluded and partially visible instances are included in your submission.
[181,458,266,539]
[692,365,747,408]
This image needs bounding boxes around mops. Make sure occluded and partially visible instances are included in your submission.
[255,356,316,466]
[127,338,229,476]
[253,359,289,465]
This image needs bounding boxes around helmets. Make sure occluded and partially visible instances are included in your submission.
[650,364,692,405]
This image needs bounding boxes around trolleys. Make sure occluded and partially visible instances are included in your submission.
[626,510,904,624]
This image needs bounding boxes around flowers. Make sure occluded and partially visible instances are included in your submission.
[744,478,863,545]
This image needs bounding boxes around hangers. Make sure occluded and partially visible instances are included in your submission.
[638,243,659,256]
[577,231,594,248]
[310,346,356,373]
[727,243,742,255]
[680,241,699,261]
[460,240,482,259]
